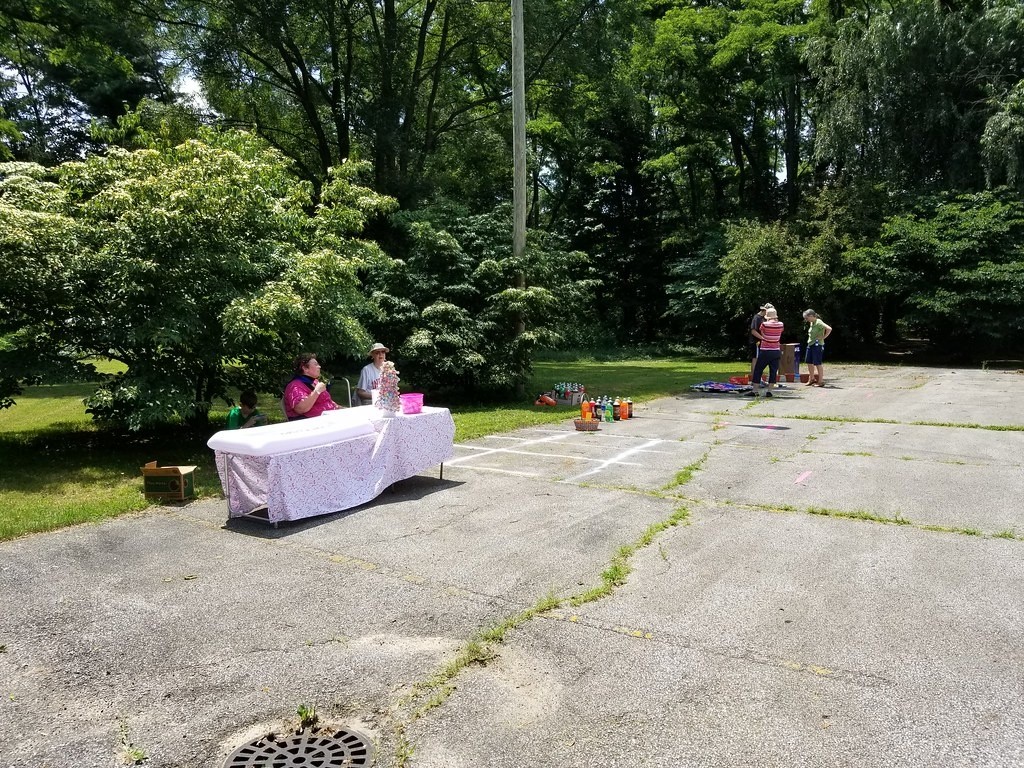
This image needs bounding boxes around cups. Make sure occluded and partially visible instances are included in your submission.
[585,412,592,420]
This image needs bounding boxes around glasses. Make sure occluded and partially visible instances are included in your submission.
[376,350,385,355]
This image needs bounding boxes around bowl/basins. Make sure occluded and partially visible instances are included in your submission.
[762,375,766,381]
[786,375,794,382]
[748,375,753,381]
[776,375,780,382]
[800,374,819,383]
[737,377,749,385]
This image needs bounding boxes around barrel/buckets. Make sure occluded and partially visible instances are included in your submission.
[400,393,424,414]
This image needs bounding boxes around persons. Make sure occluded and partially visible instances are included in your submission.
[284,352,344,421]
[749,303,774,388]
[802,309,833,387]
[227,389,271,430]
[743,308,784,397]
[356,343,389,405]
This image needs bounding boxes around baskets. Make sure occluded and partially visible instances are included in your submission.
[573,393,600,431]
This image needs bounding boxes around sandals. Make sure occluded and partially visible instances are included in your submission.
[805,378,817,386]
[815,381,825,386]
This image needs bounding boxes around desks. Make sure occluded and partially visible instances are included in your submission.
[207,403,456,528]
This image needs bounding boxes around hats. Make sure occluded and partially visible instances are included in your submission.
[764,308,778,319]
[369,343,389,356]
[760,303,774,311]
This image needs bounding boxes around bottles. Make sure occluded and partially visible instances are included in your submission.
[533,382,585,405]
[581,396,632,422]
[815,339,818,346]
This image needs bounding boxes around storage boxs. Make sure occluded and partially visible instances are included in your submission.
[552,390,583,406]
[140,461,197,500]
[777,343,799,375]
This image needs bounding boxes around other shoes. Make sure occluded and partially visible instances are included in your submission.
[751,382,765,388]
[744,391,759,397]
[766,392,773,397]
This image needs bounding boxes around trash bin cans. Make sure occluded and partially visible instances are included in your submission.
[778,343,800,376]
